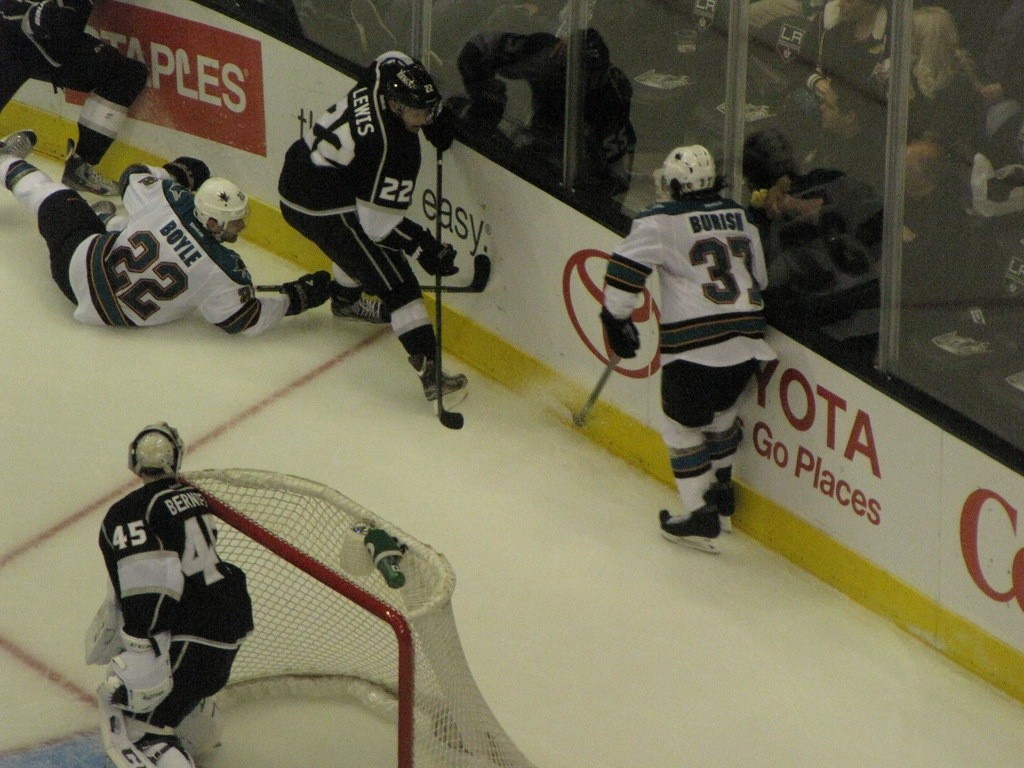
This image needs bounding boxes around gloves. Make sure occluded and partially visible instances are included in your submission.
[420,93,455,151]
[163,157,210,192]
[29,0,79,40]
[405,229,459,276]
[280,271,336,316]
[601,305,640,359]
[476,79,507,129]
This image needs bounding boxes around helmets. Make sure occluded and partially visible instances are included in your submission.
[127,422,184,477]
[743,128,791,182]
[390,64,438,109]
[662,144,715,195]
[193,177,249,235]
[586,27,610,68]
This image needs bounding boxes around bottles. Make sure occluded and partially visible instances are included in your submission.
[556,0,596,38]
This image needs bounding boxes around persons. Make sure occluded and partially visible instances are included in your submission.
[0,0,147,196]
[85,422,254,768]
[224,0,762,238]
[762,0,1024,460]
[460,29,635,238]
[0,129,336,337]
[599,145,777,554]
[740,132,884,380]
[192,1,303,50]
[278,51,469,415]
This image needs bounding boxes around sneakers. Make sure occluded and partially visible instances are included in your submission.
[660,484,720,554]
[409,353,468,413]
[92,201,116,214]
[0,130,37,158]
[332,278,391,324]
[63,138,121,195]
[715,464,735,533]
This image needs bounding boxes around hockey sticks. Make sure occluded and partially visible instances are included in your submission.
[253,255,491,294]
[529,351,620,427]
[435,146,464,430]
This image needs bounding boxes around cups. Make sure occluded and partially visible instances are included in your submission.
[676,30,696,53]
